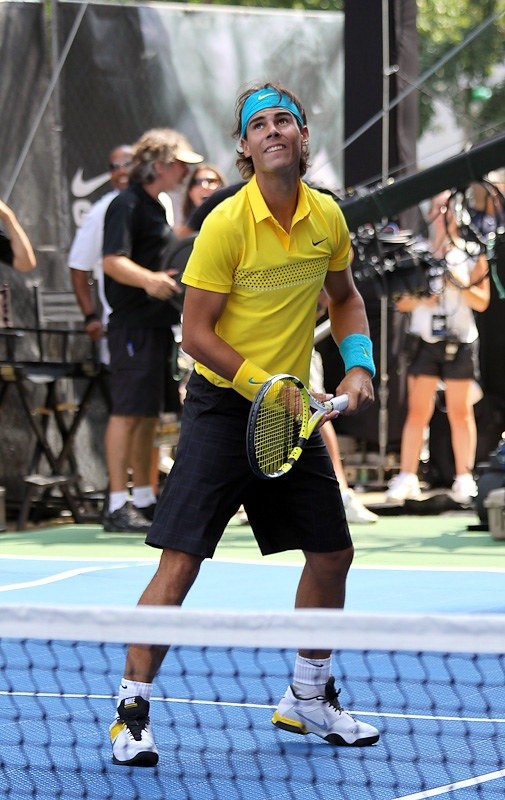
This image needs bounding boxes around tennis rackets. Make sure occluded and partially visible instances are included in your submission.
[244,372,349,482]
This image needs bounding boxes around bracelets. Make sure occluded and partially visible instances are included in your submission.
[338,334,376,379]
[86,314,98,323]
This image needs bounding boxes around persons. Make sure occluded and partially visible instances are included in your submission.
[387,169,505,505]
[66,128,226,534]
[0,197,38,273]
[109,81,380,767]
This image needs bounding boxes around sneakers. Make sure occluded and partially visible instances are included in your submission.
[109,695,160,766]
[272,676,380,746]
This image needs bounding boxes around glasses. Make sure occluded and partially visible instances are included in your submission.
[110,160,138,171]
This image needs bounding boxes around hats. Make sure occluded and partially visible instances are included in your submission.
[142,144,204,164]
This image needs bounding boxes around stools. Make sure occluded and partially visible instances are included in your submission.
[1,326,109,524]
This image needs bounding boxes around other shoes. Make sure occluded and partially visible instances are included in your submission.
[384,472,421,502]
[452,474,477,505]
[104,501,155,533]
